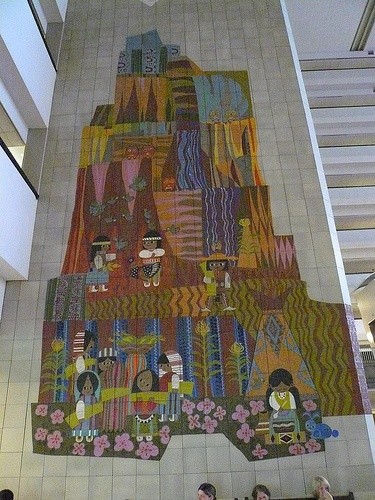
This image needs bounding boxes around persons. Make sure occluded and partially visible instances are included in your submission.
[310,474,335,500]
[0,489,15,500]
[251,483,271,500]
[197,483,218,500]
